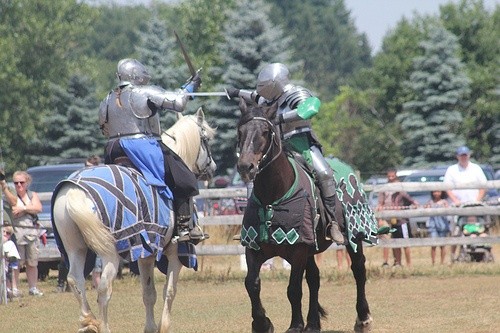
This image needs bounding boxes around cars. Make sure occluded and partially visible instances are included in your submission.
[15,162,85,280]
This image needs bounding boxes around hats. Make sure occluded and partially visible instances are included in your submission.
[457,147,469,155]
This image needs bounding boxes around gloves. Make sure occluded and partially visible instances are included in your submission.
[273,113,284,125]
[227,87,240,98]
[182,67,204,100]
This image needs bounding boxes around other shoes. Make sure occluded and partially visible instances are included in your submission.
[181,230,209,242]
[328,221,345,244]
[29,288,44,296]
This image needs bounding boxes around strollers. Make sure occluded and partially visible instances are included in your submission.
[456,203,496,263]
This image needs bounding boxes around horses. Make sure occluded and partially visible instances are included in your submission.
[52,107,217,333]
[237,96,371,333]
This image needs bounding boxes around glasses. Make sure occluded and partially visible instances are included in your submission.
[14,181,25,184]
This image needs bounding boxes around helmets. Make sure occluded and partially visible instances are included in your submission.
[256,63,290,100]
[116,58,148,86]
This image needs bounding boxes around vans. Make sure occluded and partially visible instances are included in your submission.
[362,162,500,232]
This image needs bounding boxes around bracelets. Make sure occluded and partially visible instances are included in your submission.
[23,206,26,211]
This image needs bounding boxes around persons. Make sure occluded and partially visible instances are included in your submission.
[84,155,103,288]
[462,216,487,251]
[425,191,450,266]
[336,243,350,270]
[378,164,419,267]
[0,169,44,296]
[100,59,209,243]
[443,146,487,266]
[225,62,345,244]
[1,223,21,273]
